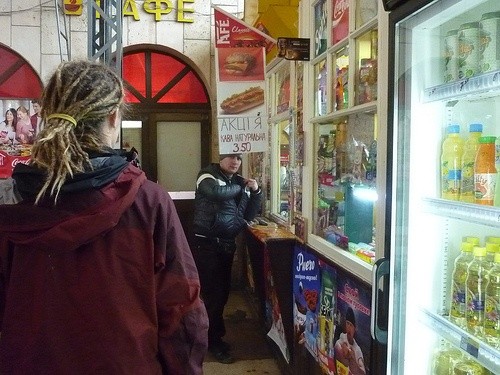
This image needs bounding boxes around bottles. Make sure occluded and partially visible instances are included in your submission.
[441,125,463,200]
[460,124,483,201]
[449,236,500,348]
[493,138,500,207]
[474,137,496,205]
[317,129,337,176]
[432,339,494,375]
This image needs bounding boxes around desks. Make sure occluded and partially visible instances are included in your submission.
[247,224,295,362]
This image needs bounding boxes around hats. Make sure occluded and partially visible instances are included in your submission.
[346,307,356,326]
[218,153,243,162]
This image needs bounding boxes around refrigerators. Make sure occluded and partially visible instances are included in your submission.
[370,0,500,375]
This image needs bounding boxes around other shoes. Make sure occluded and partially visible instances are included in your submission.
[208,340,234,364]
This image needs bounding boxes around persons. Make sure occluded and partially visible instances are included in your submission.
[334,308,367,375]
[0,98,45,144]
[0,59,210,375]
[189,152,264,363]
